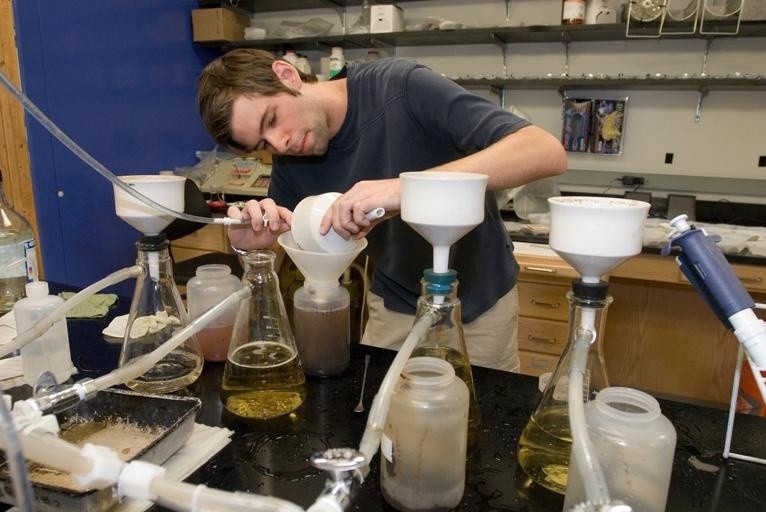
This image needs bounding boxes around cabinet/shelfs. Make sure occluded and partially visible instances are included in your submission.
[516,255,580,376]
[168,211,288,308]
[189,2,766,118]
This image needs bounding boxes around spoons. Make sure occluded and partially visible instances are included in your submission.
[354,353,372,413]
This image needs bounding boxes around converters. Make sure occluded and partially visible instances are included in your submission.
[621,175,644,185]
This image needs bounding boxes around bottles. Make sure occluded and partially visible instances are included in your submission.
[118,237,205,395]
[563,385,677,512]
[0,169,41,317]
[328,45,345,80]
[561,1,586,25]
[184,250,352,419]
[367,51,378,61]
[378,357,470,511]
[281,49,311,75]
[14,283,73,389]
[411,269,487,437]
[519,295,617,498]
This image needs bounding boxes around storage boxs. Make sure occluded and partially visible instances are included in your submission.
[190,9,252,43]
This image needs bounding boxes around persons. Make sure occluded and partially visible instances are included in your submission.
[193,47,569,380]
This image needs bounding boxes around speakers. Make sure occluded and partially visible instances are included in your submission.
[371,3,403,33]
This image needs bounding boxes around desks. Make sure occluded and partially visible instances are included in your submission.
[603,251,766,420]
[1,279,765,512]
